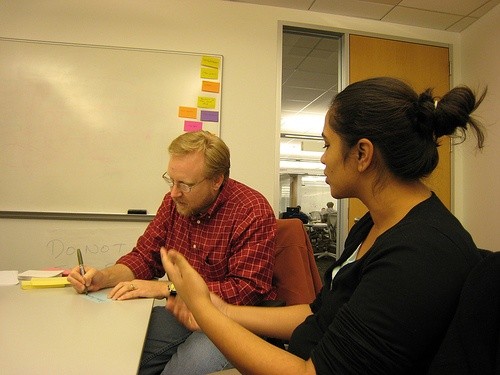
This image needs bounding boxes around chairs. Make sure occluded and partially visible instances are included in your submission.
[435,248,500,375]
[313,214,337,262]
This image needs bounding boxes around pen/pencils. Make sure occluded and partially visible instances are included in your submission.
[77,249,88,295]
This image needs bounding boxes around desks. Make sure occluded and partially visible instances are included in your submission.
[303,223,327,228]
[0,278,159,375]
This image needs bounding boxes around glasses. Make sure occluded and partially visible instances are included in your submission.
[162,172,214,192]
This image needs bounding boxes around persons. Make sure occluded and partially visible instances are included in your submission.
[67,130,283,375]
[322,202,336,215]
[160,77,500,375]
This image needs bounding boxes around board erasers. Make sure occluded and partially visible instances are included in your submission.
[128,209,147,214]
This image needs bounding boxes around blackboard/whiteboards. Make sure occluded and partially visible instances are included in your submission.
[0,36,224,222]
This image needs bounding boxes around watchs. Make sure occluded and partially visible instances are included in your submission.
[167,281,177,299]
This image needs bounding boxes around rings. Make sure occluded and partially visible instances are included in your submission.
[130,284,135,290]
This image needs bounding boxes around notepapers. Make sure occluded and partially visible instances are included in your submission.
[21,277,71,289]
[19,270,63,280]
[44,268,72,276]
